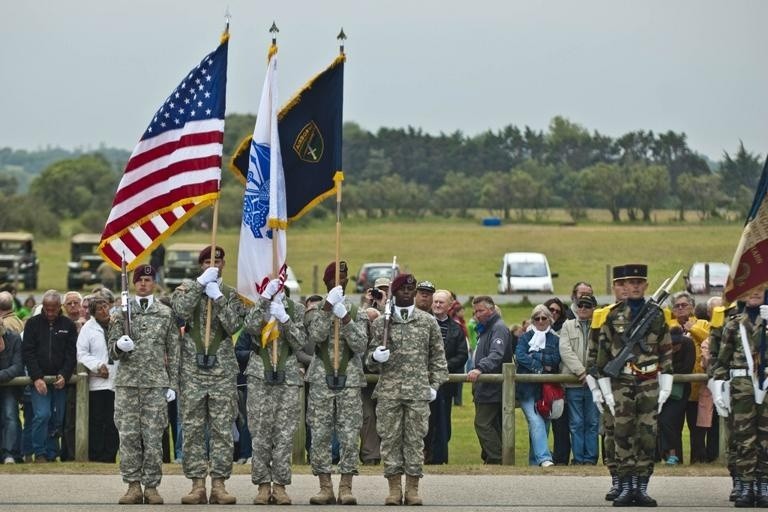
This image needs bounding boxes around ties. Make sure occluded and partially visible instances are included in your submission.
[400,309,408,321]
[139,299,148,309]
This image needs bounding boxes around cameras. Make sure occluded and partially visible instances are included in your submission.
[370,290,381,299]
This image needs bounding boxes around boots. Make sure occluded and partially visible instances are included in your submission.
[310,473,337,504]
[633,477,657,507]
[181,478,208,504]
[630,476,638,506]
[272,482,292,505]
[209,479,236,504]
[734,480,756,507]
[606,475,622,501]
[337,473,358,505]
[757,480,768,508]
[119,481,143,505]
[728,476,742,500]
[404,474,423,506]
[752,480,758,504]
[253,483,272,505]
[614,480,633,507]
[143,484,164,504]
[384,474,403,506]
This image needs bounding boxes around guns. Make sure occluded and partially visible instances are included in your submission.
[378,254,398,370]
[601,267,685,379]
[120,250,133,355]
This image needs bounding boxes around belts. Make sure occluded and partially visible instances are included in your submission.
[621,362,659,375]
[729,368,768,377]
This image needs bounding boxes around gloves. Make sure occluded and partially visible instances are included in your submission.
[270,299,290,324]
[196,267,218,287]
[585,374,605,414]
[206,282,224,303]
[428,387,437,404]
[759,304,768,321]
[597,377,616,416]
[372,346,390,364]
[333,303,348,319]
[165,389,177,402]
[260,279,280,300]
[326,285,343,306]
[657,371,673,415]
[116,334,134,353]
[706,378,729,417]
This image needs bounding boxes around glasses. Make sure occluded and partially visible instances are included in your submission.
[672,303,692,308]
[577,304,593,309]
[65,301,79,305]
[549,308,561,314]
[534,317,547,321]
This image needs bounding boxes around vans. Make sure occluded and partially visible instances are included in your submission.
[495,251,558,294]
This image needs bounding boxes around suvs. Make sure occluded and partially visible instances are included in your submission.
[0,231,37,291]
[68,233,122,290]
[163,242,210,291]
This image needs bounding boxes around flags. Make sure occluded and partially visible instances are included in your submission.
[722,155,768,306]
[98,32,228,271]
[229,53,346,221]
[237,46,288,349]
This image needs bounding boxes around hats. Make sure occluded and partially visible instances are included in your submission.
[623,264,648,279]
[323,260,348,281]
[132,265,155,284]
[391,273,416,296]
[579,295,597,306]
[416,281,435,293]
[374,277,391,288]
[612,266,624,281]
[198,245,225,264]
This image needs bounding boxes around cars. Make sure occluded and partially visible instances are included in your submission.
[350,262,402,294]
[681,260,731,293]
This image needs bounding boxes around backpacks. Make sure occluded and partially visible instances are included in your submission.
[535,374,566,420]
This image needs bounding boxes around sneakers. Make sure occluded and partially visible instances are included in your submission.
[667,455,679,466]
[35,457,48,463]
[4,457,16,465]
[540,461,555,468]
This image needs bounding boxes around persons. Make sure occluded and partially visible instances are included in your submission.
[297,262,369,504]
[587,264,674,506]
[365,274,448,505]
[0,280,768,466]
[240,271,307,504]
[170,245,247,504]
[707,277,768,508]
[106,262,181,506]
